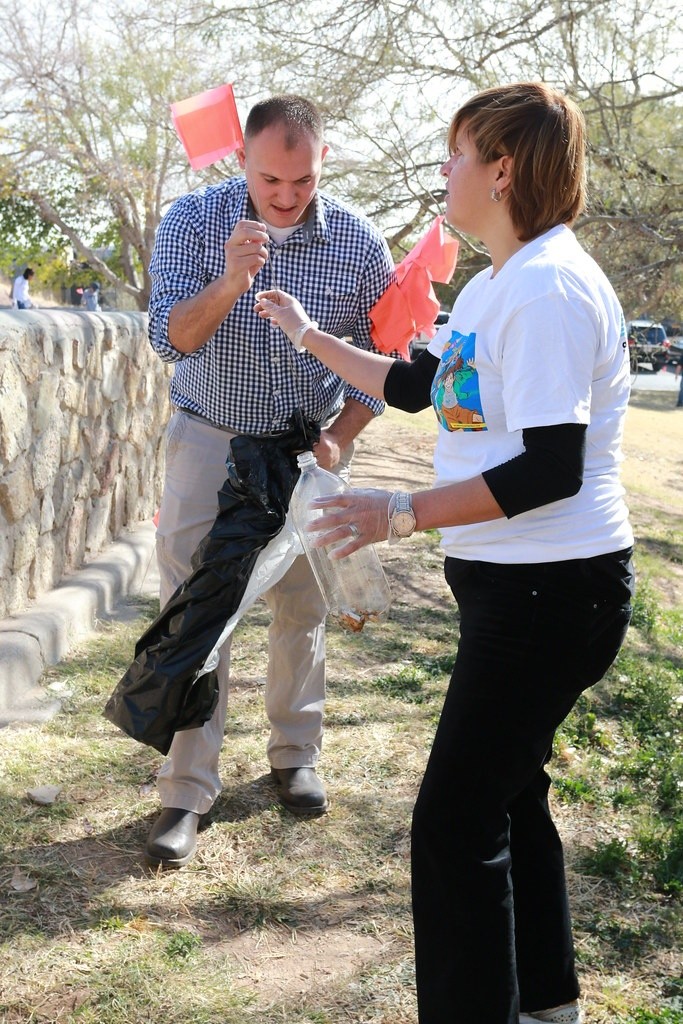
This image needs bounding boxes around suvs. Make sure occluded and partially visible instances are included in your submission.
[409,310,450,359]
[627,320,670,374]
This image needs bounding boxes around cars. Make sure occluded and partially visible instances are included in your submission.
[663,322,683,366]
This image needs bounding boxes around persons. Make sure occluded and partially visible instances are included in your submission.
[145,94,416,868]
[81,282,100,312]
[12,267,35,310]
[674,353,683,408]
[250,83,637,1024]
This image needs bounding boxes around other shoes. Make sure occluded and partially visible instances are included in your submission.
[145,807,199,866]
[520,1015,581,1024]
[271,765,327,813]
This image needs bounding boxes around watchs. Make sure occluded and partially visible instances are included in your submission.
[389,491,418,540]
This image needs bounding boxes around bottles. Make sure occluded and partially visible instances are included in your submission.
[292,453,388,615]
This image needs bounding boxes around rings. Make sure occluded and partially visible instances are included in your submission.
[348,523,359,538]
